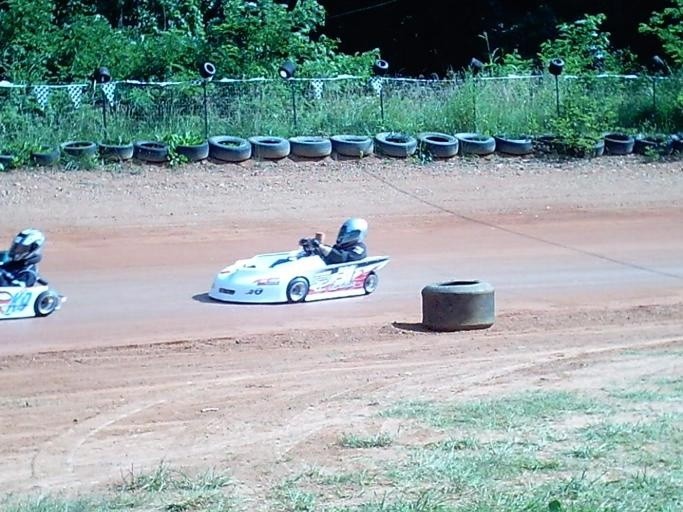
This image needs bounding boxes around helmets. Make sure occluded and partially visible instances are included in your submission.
[10,228,45,266]
[335,217,368,248]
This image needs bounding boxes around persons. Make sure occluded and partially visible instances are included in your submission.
[297,217,370,264]
[0,229,45,288]
